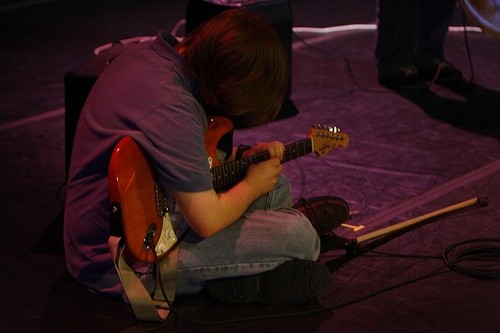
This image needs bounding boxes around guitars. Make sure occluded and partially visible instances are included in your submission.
[107,113,352,265]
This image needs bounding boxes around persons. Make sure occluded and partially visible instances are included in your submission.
[60,10,349,308]
[373,0,466,90]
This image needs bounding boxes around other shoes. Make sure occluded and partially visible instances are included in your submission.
[292,196,349,234]
[207,260,330,304]
[379,72,437,90]
[428,57,462,82]
